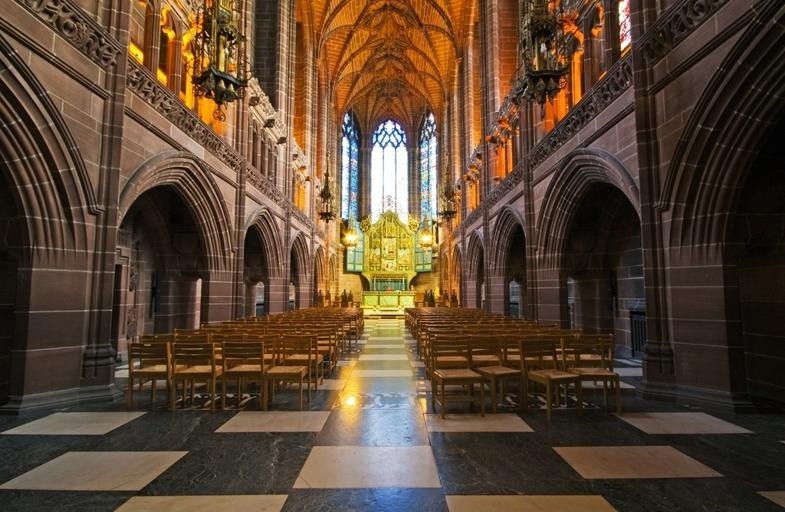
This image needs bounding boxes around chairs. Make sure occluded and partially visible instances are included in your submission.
[405,305,622,424]
[127,304,364,411]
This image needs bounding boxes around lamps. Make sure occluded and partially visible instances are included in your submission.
[437,0,457,223]
[510,0,574,122]
[317,44,336,224]
[343,57,358,251]
[184,0,258,122]
[418,58,434,247]
